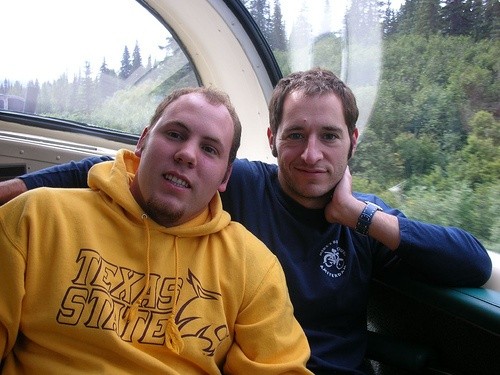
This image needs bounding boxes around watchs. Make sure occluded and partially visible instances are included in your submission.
[355,201,383,237]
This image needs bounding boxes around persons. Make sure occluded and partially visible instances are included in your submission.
[0,66,492,375]
[0,88,312,375]
